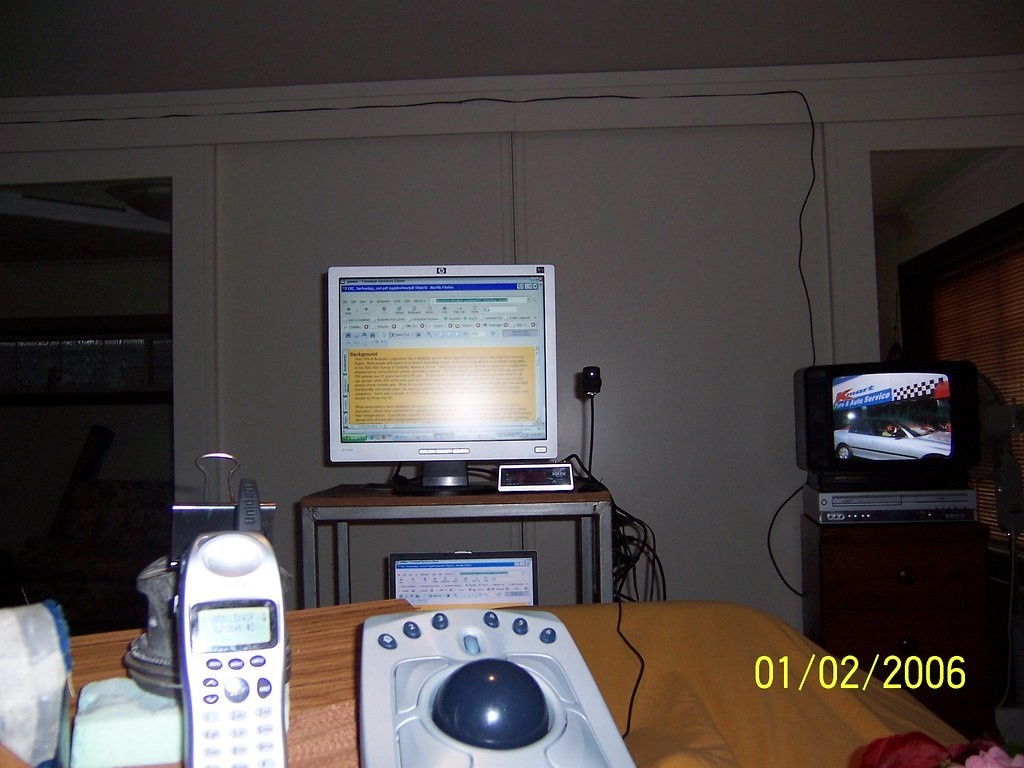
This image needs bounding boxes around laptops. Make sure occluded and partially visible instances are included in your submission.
[388,550,538,606]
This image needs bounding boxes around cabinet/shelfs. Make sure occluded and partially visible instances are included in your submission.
[800,512,990,665]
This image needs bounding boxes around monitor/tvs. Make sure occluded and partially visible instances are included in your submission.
[793,360,977,493]
[327,265,556,499]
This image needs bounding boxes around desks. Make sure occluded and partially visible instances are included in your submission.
[300,477,613,609]
[0,599,414,768]
